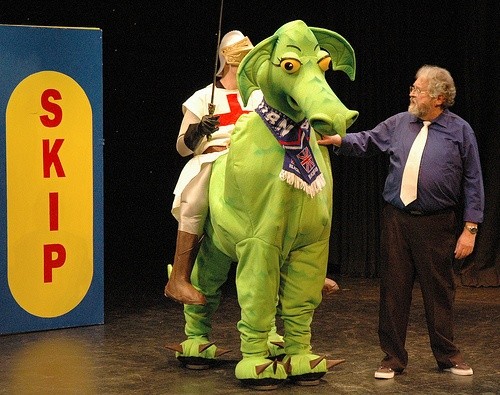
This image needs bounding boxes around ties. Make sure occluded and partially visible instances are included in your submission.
[400,120,431,207]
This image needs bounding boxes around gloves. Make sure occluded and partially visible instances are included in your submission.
[184,113,220,151]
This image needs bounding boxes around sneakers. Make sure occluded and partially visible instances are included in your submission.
[374,364,399,380]
[445,365,473,376]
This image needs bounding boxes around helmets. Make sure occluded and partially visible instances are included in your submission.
[216,30,246,77]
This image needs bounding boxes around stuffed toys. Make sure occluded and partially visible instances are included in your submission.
[167,20,359,381]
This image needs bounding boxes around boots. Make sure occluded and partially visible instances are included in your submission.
[164,229,205,306]
[319,277,338,295]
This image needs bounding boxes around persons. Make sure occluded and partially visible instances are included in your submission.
[318,65,485,379]
[177,30,264,157]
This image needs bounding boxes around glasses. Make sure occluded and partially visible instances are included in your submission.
[409,86,431,94]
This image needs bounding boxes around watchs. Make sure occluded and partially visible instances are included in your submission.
[465,225,478,234]
